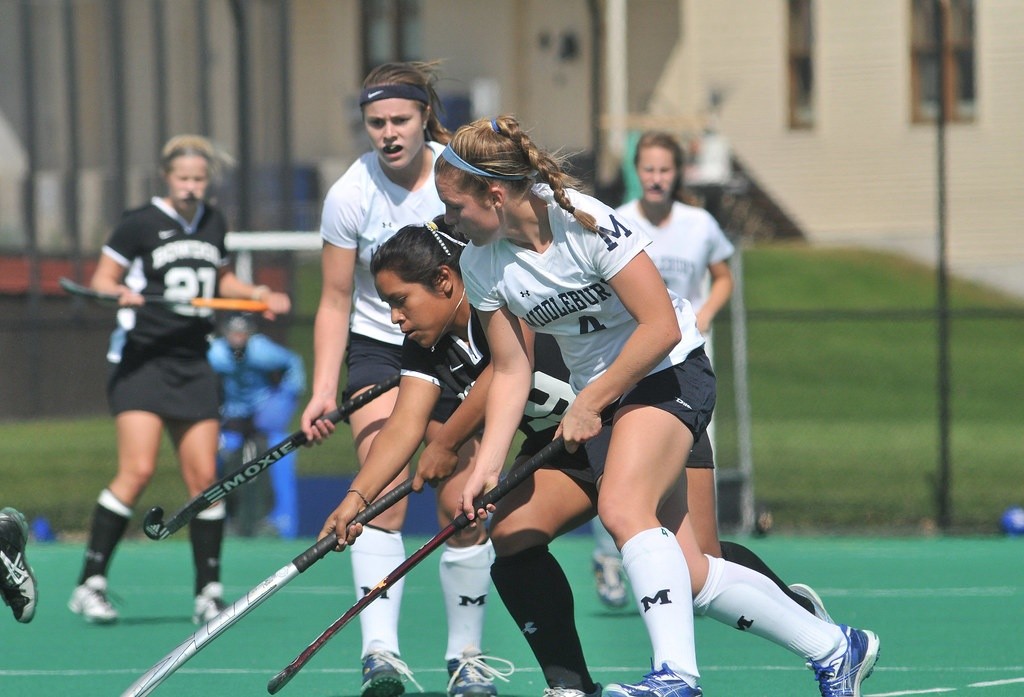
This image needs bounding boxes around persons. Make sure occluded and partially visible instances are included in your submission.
[71,136,291,624]
[0,507,39,625]
[208,61,880,697]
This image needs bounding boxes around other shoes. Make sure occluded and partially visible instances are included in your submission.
[787,583,835,625]
[191,582,227,623]
[592,548,627,608]
[66,575,125,625]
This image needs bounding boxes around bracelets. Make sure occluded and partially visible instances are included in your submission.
[251,284,271,301]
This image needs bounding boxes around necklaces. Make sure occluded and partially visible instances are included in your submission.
[430,289,466,351]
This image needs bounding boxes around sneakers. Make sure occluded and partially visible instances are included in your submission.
[360,640,425,697]
[0,507,37,623]
[444,647,515,697]
[542,682,603,697]
[805,624,880,697]
[601,656,702,697]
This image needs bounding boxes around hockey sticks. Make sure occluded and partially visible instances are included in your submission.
[117,473,416,697]
[57,271,268,313]
[266,431,567,696]
[142,371,400,541]
